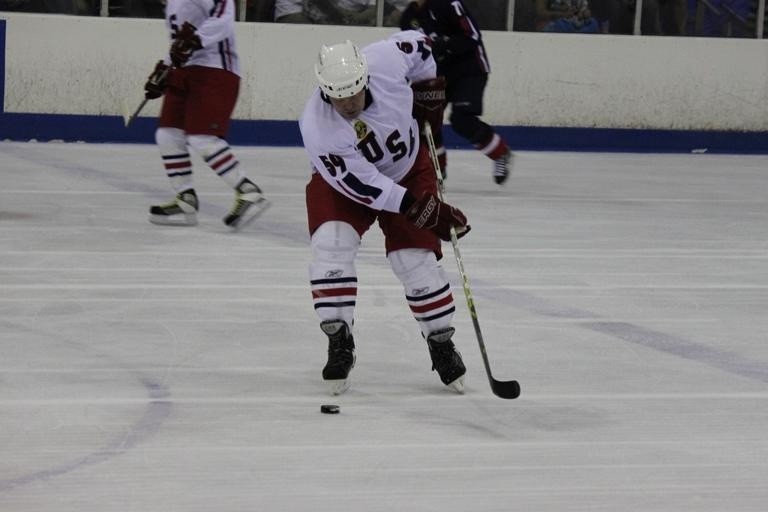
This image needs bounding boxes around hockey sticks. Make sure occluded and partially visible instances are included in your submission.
[120,63,174,128]
[423,120,520,399]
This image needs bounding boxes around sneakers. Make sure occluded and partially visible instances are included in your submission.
[222,176,262,225]
[492,149,510,184]
[150,189,197,214]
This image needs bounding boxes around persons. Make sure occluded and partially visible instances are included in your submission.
[399,0,512,188]
[144,0,263,226]
[294,29,469,386]
[266,1,767,46]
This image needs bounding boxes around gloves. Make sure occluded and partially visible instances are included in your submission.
[403,190,470,242]
[170,35,201,63]
[409,77,446,136]
[145,64,170,98]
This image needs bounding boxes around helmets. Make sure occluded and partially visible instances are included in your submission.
[314,39,369,98]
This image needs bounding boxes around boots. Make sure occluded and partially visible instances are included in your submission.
[319,319,356,380]
[420,327,465,385]
